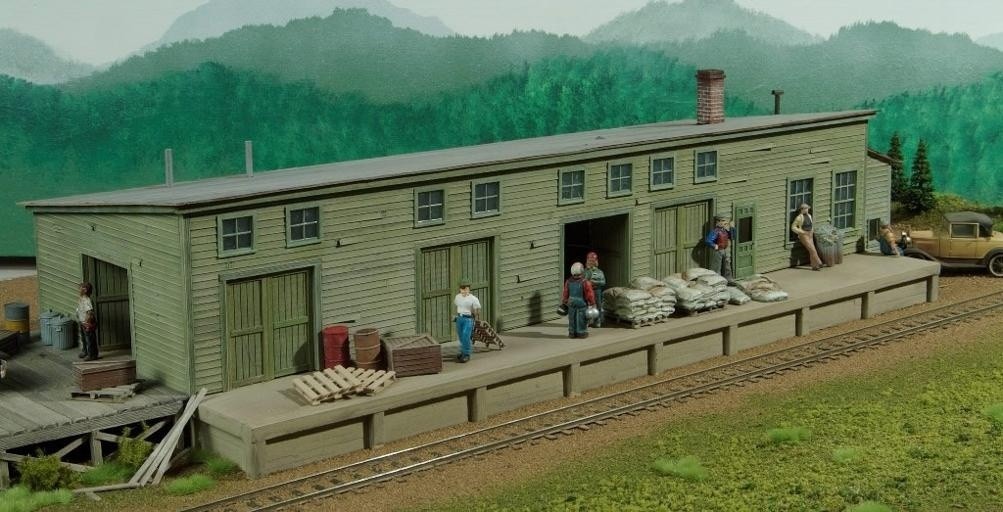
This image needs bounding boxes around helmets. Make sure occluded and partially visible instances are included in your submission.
[570,261,584,275]
[586,251,599,262]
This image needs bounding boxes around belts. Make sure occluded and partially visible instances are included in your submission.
[457,312,472,318]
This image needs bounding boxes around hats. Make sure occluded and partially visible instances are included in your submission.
[459,280,470,288]
[714,214,729,220]
[879,218,889,226]
[798,203,811,209]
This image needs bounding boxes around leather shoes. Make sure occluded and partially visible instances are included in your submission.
[812,263,826,271]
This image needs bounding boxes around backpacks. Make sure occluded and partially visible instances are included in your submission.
[879,231,893,255]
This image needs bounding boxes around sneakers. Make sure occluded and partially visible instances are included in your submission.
[588,321,601,328]
[567,331,588,339]
[456,353,469,363]
[78,352,97,361]
[726,281,738,287]
